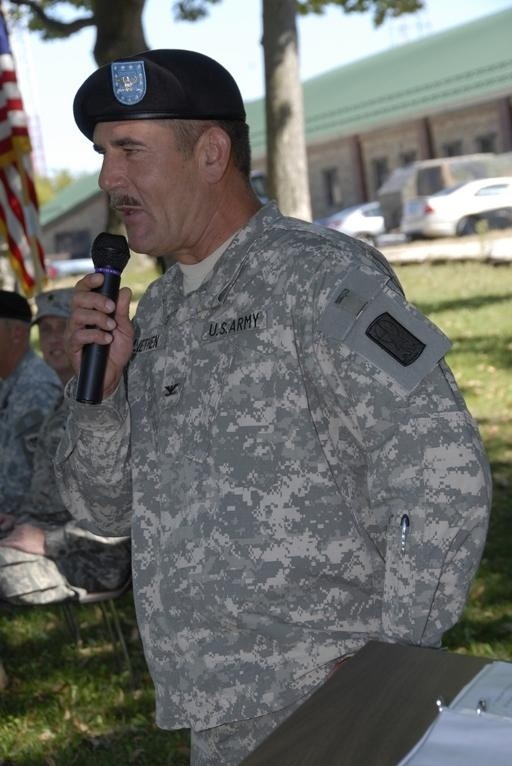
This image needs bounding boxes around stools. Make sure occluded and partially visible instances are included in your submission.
[60,582,136,677]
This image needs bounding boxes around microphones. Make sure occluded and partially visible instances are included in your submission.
[78,233,131,405]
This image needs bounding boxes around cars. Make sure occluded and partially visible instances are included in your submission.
[314,151,511,246]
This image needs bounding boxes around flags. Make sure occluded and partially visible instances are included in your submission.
[0,10,51,296]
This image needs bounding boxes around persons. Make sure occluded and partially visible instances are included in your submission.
[0,286,132,692]
[0,290,62,543]
[51,48,494,765]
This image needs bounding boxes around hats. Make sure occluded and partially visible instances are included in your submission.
[72,49,245,142]
[29,288,77,327]
[0,290,32,322]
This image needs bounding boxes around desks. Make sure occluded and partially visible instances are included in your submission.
[236,641,512,766]
